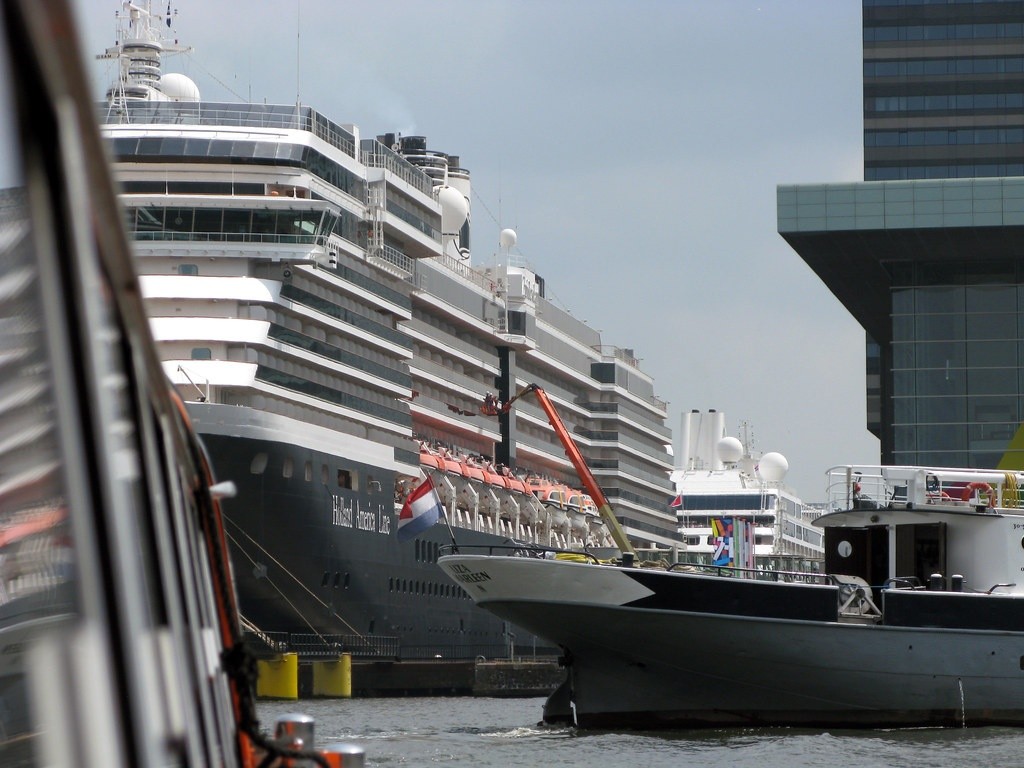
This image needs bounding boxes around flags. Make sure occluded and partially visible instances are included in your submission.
[669,493,684,508]
[397,471,447,544]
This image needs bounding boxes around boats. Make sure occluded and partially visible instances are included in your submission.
[413,440,604,541]
[434,463,1024,730]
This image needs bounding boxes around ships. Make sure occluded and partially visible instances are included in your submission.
[87,4,684,692]
[669,408,827,584]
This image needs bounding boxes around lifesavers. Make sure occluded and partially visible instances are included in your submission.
[942,492,951,501]
[962,481,995,510]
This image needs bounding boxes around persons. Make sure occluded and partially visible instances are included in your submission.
[485,391,499,411]
